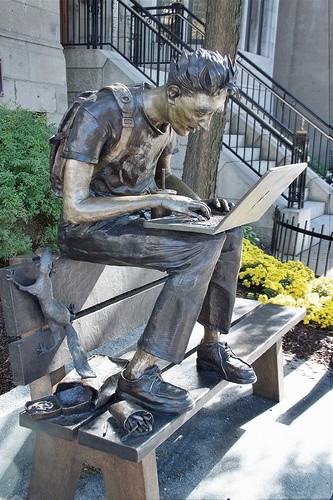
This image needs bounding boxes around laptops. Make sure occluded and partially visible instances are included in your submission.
[144,162,307,235]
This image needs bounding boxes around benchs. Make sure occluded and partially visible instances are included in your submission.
[0,255,306,500]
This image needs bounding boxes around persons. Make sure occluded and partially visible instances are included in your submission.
[48,50,257,414]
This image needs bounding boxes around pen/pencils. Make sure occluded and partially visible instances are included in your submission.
[102,423,107,437]
[119,414,153,441]
